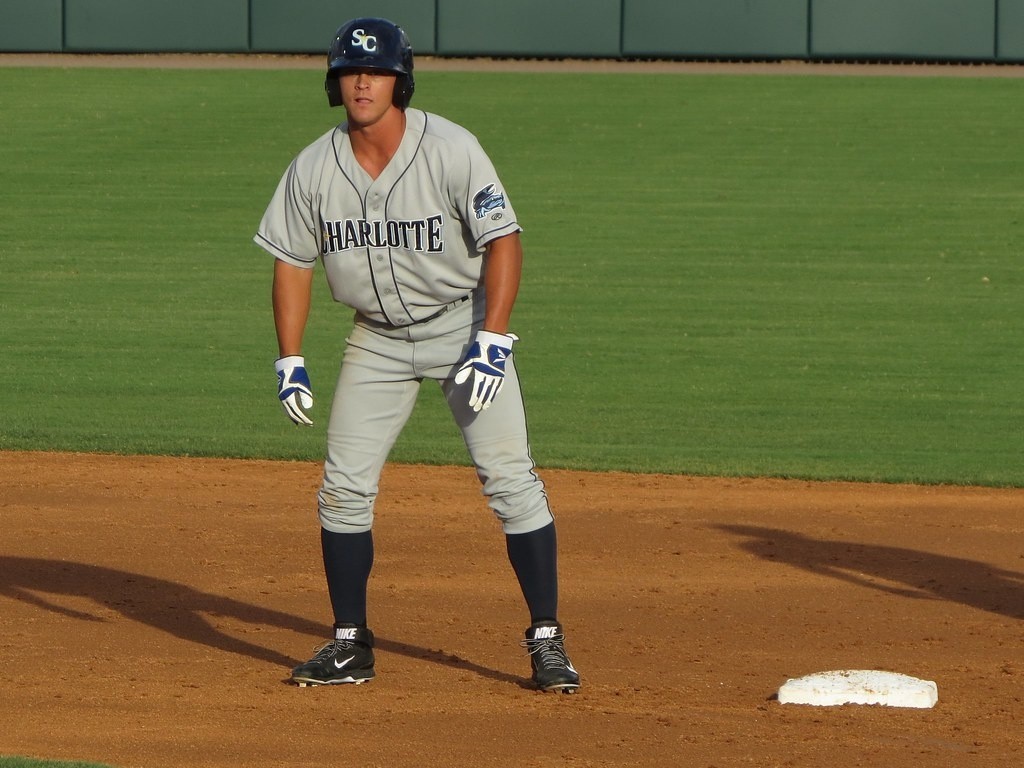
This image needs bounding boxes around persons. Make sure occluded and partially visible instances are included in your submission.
[252,16,580,693]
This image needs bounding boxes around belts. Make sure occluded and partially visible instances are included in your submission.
[416,296,469,323]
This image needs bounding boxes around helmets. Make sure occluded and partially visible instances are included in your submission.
[324,17,415,113]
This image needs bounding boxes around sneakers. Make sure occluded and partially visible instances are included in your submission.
[518,620,581,694]
[291,622,376,688]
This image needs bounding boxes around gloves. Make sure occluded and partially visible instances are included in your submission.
[272,355,314,427]
[453,328,520,413]
